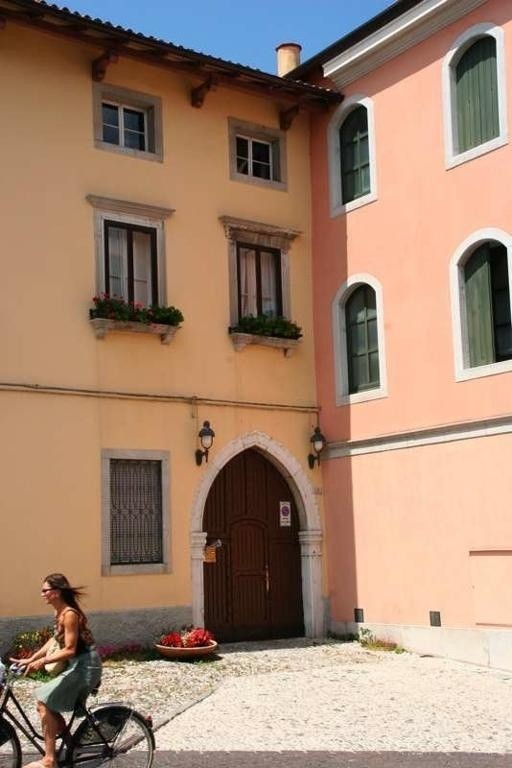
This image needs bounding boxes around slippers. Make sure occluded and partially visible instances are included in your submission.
[22,760,48,767]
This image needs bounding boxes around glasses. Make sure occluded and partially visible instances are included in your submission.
[40,587,58,593]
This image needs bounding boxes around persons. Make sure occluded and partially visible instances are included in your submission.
[8,572,104,768]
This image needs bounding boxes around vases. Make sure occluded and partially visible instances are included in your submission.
[151,639,218,657]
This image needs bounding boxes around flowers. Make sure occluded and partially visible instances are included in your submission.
[90,290,184,326]
[159,623,213,647]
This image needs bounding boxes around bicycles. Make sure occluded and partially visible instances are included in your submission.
[0,651,157,767]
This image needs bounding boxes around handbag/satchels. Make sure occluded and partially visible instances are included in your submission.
[42,638,68,674]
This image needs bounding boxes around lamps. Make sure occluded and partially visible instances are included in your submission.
[195,419,215,467]
[308,426,326,469]
